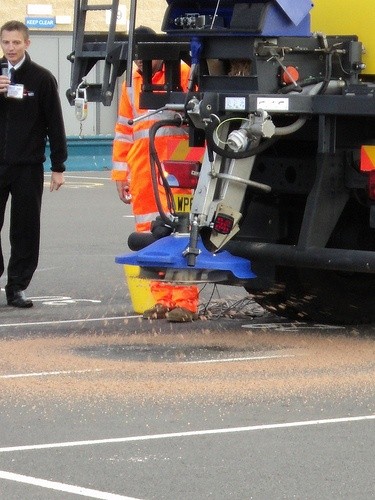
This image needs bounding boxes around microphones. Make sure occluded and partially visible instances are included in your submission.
[2,63,9,98]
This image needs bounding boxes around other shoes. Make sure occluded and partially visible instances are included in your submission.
[142,301,199,323]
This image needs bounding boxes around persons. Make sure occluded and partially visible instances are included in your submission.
[110,26,199,323]
[0,21,68,308]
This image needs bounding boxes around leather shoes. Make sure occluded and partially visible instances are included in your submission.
[7,291,33,308]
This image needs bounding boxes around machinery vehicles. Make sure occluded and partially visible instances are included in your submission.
[64,0,375,325]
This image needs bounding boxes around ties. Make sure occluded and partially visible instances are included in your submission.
[9,67,17,85]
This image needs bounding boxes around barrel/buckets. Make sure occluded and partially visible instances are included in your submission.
[123,265,156,316]
